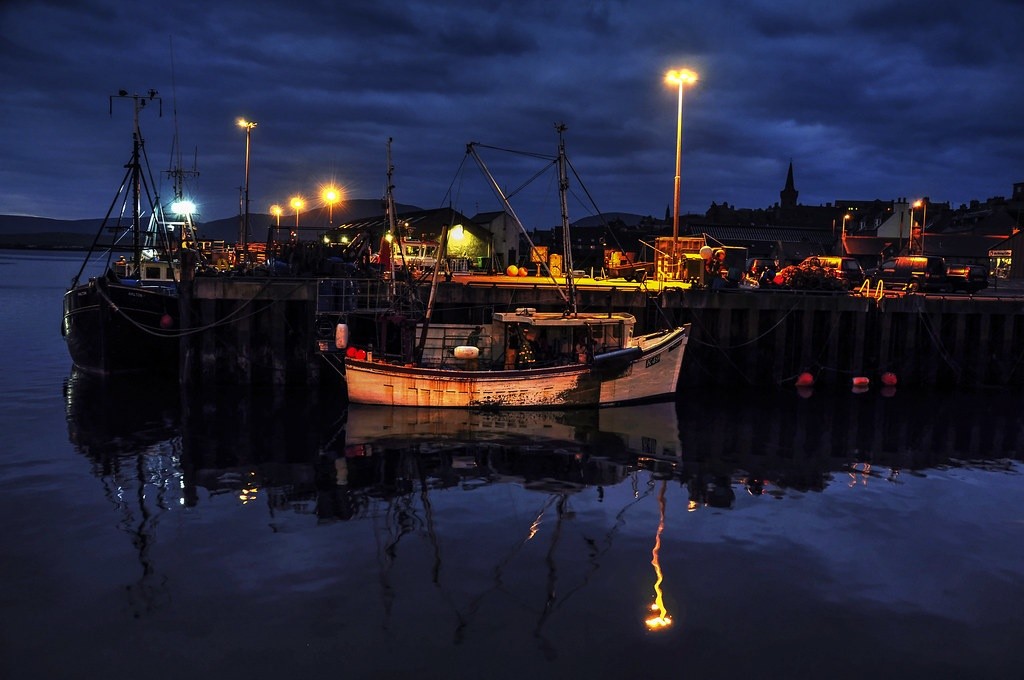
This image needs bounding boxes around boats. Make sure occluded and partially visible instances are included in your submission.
[342,400,685,484]
[60,87,692,406]
[63,364,184,588]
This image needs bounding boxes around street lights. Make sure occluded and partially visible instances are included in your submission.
[662,62,700,256]
[286,193,308,244]
[271,204,285,241]
[841,214,851,256]
[235,117,257,272]
[318,181,346,224]
[888,208,903,254]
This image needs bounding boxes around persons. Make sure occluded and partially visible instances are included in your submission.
[466,326,483,345]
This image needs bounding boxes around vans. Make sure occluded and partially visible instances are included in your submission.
[798,255,865,290]
[863,256,947,294]
[946,264,989,294]
